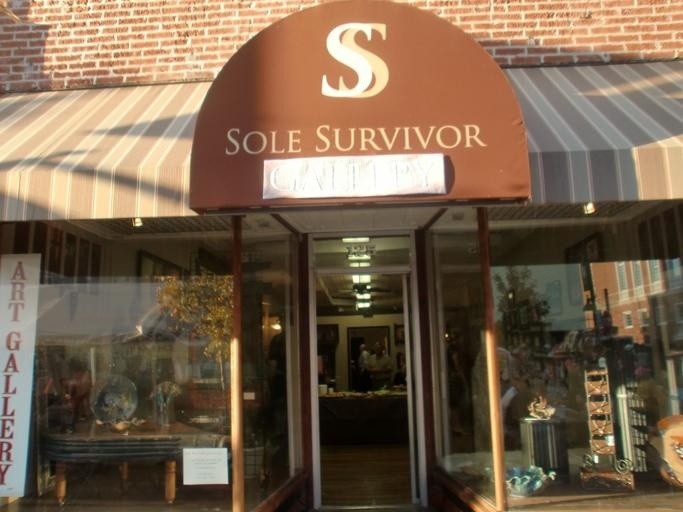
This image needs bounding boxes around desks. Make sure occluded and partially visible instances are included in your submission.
[36,421,218,507]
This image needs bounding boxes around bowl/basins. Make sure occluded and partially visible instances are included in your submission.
[489,465,558,498]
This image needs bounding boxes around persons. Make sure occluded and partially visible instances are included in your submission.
[464,316,516,452]
[355,344,368,372]
[363,341,391,389]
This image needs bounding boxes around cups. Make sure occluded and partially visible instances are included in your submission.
[318,384,328,395]
[329,388,334,394]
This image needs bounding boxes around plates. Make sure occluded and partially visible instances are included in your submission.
[88,375,138,424]
[324,391,407,399]
